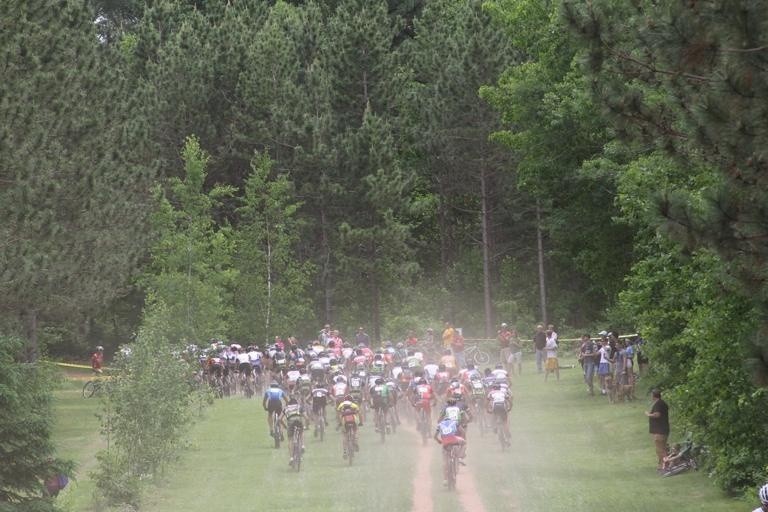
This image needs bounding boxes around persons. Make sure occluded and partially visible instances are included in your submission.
[41,458,60,498]
[170,323,522,486]
[90,345,105,375]
[577,331,650,405]
[533,325,548,372]
[751,484,768,512]
[544,330,560,383]
[545,325,558,346]
[645,389,670,469]
[662,444,688,471]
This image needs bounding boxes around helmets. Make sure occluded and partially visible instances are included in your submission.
[760,484,768,503]
[270,340,503,421]
[217,341,259,354]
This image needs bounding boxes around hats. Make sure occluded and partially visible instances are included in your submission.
[598,331,607,336]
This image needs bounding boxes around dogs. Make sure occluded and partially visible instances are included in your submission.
[605,376,633,404]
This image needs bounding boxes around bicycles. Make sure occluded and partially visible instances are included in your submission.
[457,344,491,366]
[655,440,697,477]
[412,404,435,446]
[371,405,394,442]
[264,407,285,449]
[285,425,310,474]
[166,365,270,400]
[264,355,513,422]
[337,424,364,469]
[82,377,115,398]
[487,410,510,452]
[309,402,330,442]
[440,442,465,491]
